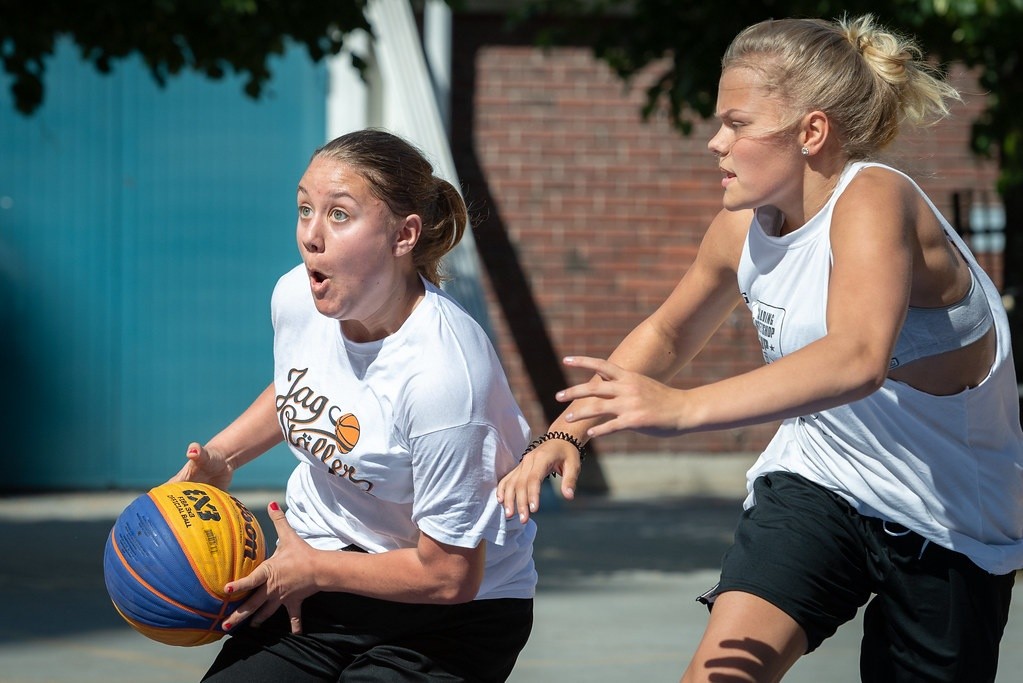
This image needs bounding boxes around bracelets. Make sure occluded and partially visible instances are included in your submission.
[519,431,586,479]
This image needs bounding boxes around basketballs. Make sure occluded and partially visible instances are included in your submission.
[102,481,269,648]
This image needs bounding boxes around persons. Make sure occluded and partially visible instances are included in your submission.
[497,19,1023,683]
[158,131,539,683]
